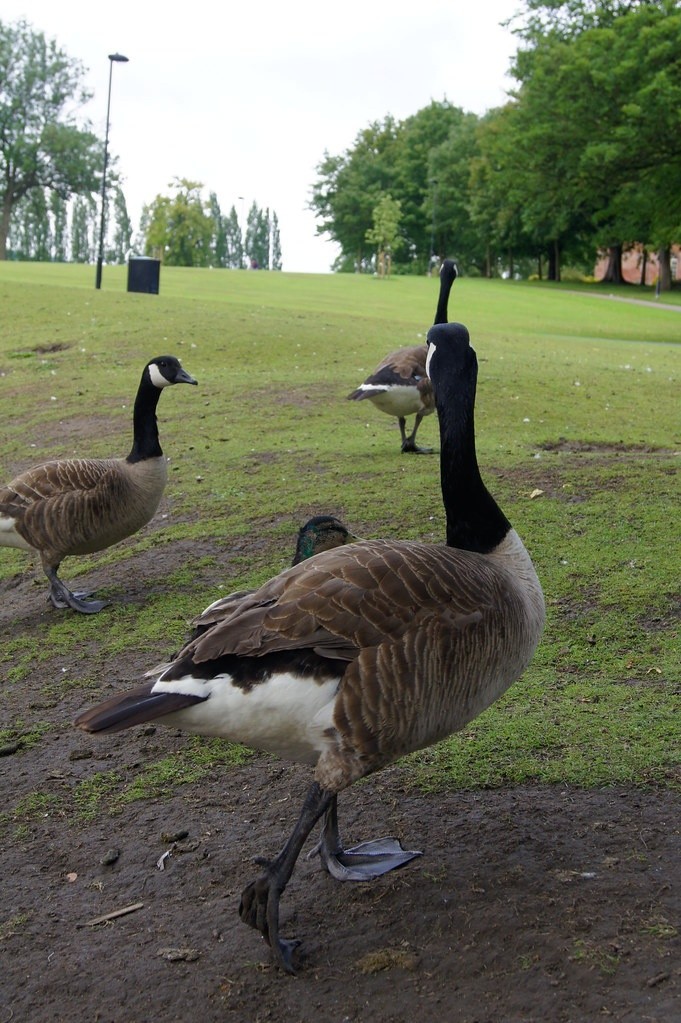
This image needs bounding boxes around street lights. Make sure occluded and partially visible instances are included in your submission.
[94,50,131,292]
[428,178,439,276]
[237,194,276,270]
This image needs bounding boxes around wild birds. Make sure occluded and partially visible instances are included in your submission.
[76,323,548,974]
[345,257,458,453]
[1,356,199,615]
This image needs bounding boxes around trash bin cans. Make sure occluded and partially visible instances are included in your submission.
[127,256,160,294]
